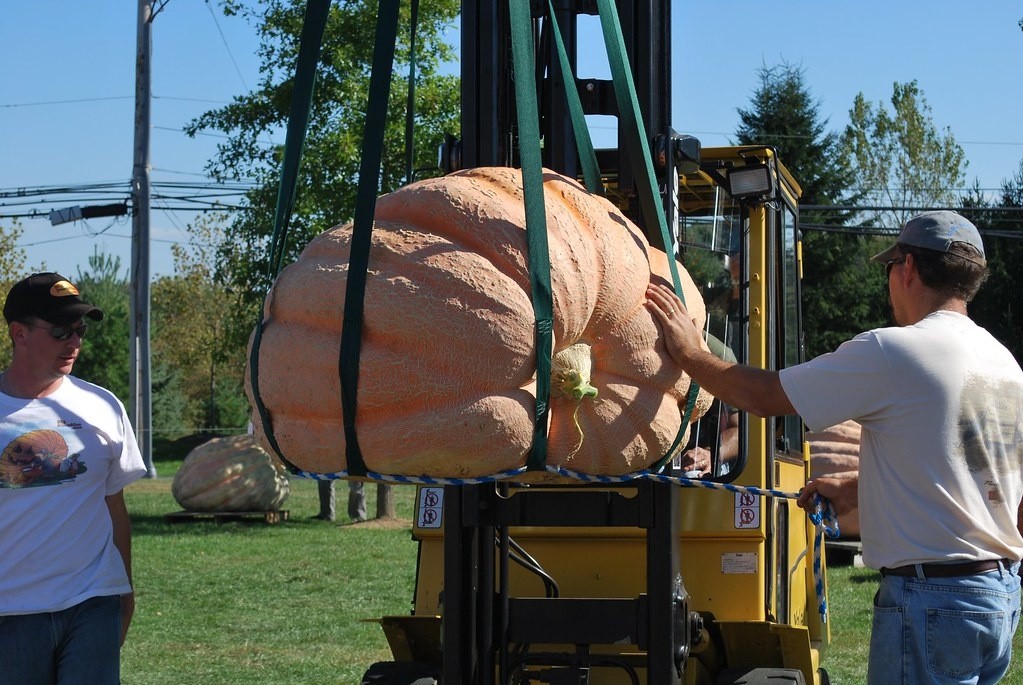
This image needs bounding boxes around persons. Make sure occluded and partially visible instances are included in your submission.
[641,207,1023,685]
[2,273,149,685]
[679,327,745,481]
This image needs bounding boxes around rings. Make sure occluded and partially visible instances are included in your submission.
[666,309,675,316]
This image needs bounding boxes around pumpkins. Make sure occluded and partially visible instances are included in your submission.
[170,164,863,540]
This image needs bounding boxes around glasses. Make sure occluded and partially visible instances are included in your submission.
[25,321,89,341]
[885,256,907,278]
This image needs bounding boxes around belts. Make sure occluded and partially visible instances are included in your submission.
[879,558,1014,579]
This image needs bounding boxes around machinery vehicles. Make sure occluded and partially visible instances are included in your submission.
[359,4,828,681]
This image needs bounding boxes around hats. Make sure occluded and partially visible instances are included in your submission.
[869,210,987,268]
[1,271,104,327]
